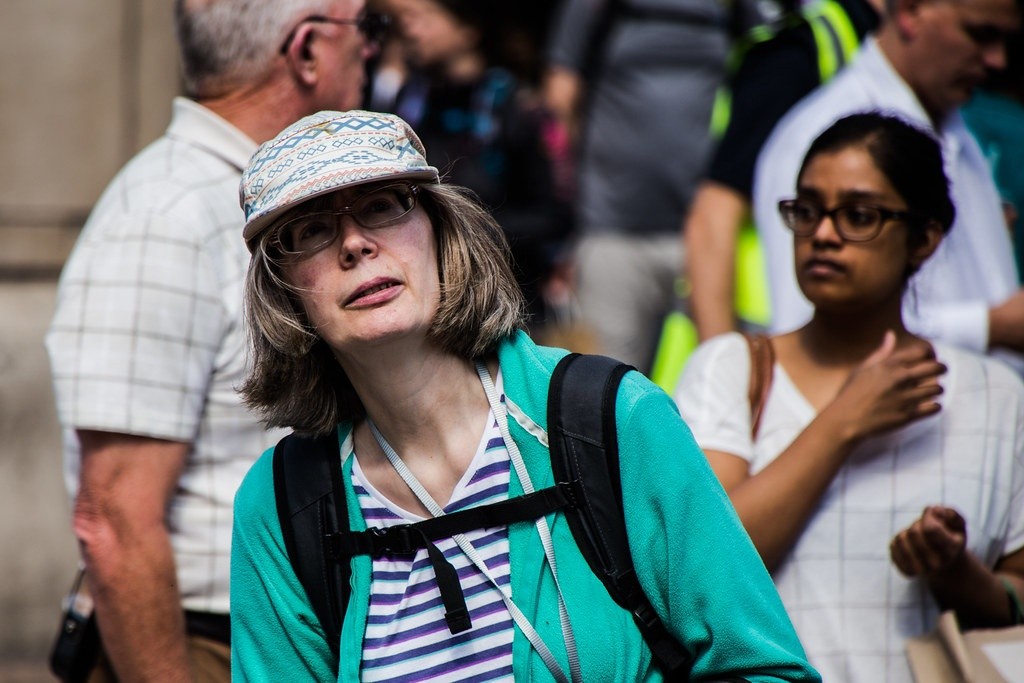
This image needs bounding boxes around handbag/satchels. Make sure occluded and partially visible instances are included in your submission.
[908,575,1024,683]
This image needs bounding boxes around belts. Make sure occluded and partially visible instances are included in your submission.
[184,611,231,646]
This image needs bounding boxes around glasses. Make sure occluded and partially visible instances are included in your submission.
[270,180,421,254]
[277,10,388,57]
[778,199,910,242]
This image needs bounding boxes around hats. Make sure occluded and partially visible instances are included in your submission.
[237,109,441,240]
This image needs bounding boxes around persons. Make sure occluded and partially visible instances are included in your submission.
[541,0,743,369]
[752,0,1024,379]
[678,109,1024,683]
[231,103,823,683]
[362,1,570,350]
[648,0,882,400]
[47,0,376,683]
